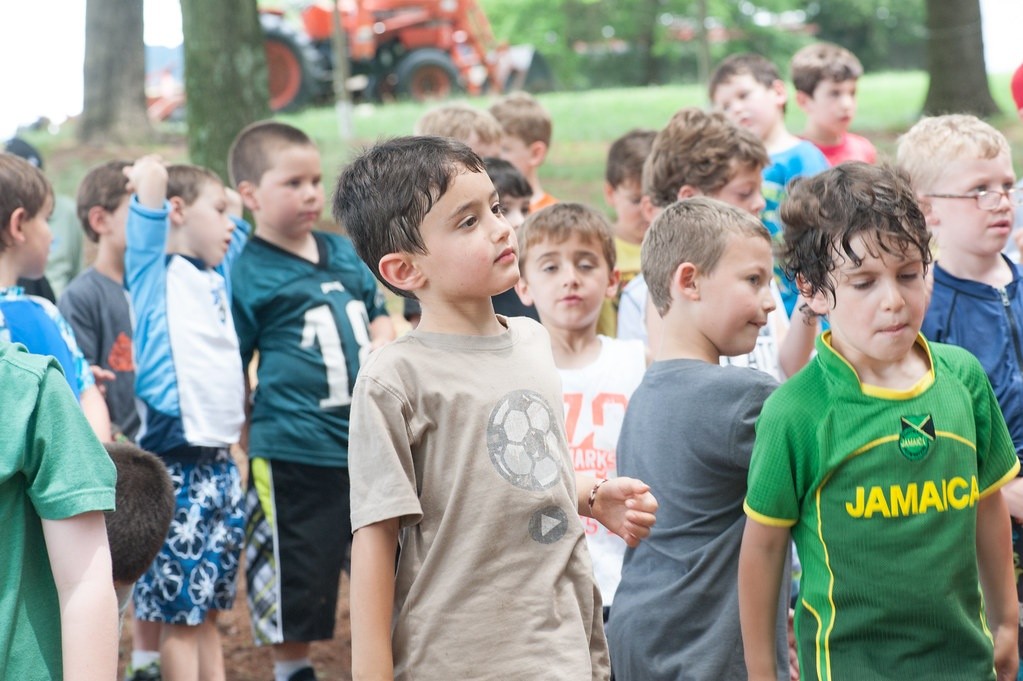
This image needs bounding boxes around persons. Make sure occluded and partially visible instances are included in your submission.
[0,43,1023,681]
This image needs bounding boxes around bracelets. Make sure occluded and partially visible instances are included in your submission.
[586,476,610,515]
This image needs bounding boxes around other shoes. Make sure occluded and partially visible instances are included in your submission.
[125,662,161,681]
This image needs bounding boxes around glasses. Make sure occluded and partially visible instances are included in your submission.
[926,188,1023,211]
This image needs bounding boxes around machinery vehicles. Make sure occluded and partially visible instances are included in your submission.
[147,0,553,118]
